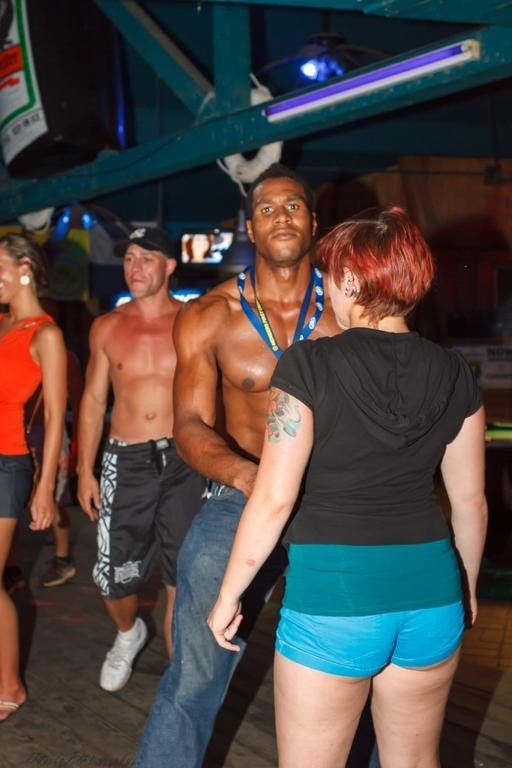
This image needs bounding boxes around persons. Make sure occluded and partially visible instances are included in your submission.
[74,221,210,698]
[205,208,495,767]
[0,232,69,727]
[3,349,87,596]
[134,162,355,767]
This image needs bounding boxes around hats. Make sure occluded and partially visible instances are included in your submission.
[111,227,176,258]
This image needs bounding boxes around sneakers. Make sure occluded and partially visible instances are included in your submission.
[3,565,26,595]
[40,562,76,587]
[99,616,149,692]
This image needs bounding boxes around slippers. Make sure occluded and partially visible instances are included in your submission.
[0,698,26,724]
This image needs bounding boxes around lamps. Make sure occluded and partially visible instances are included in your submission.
[298,12,348,80]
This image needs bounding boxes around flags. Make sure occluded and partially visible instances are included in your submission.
[2,0,51,166]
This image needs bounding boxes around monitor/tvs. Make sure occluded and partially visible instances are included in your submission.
[106,278,212,311]
[176,226,237,269]
